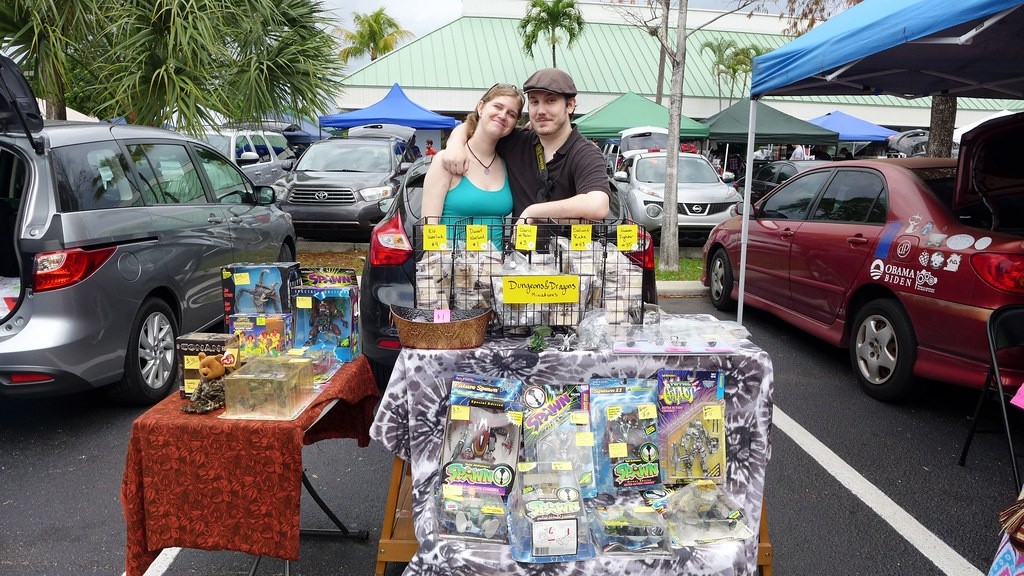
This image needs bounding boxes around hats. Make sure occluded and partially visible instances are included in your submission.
[523,68,577,98]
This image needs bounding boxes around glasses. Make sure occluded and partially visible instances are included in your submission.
[481,83,525,109]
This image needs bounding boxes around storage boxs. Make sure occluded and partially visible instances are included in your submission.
[228,313,294,363]
[223,260,301,334]
[223,358,315,416]
[176,332,242,399]
[289,260,361,362]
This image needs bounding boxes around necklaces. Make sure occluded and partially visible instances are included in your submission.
[466,139,497,175]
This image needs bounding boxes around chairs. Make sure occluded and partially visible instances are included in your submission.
[79,163,120,209]
[959,303,1024,495]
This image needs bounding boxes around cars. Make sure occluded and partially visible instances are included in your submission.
[273,124,416,239]
[700,112,1024,401]
[0,49,299,409]
[359,155,659,374]
[600,126,745,242]
[735,160,835,204]
[177,129,297,186]
[886,129,959,157]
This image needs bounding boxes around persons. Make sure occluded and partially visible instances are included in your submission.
[789,145,831,160]
[690,487,739,530]
[683,420,712,477]
[421,85,526,250]
[441,68,611,255]
[987,382,1024,576]
[409,135,422,159]
[425,140,437,155]
[303,300,348,348]
[593,139,598,145]
[840,148,854,159]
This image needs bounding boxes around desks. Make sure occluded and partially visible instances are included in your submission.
[123,346,376,576]
[375,313,776,576]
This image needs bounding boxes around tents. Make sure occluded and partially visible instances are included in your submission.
[319,83,462,140]
[697,97,839,173]
[573,92,710,172]
[952,110,1013,144]
[807,110,902,158]
[736,1,1024,327]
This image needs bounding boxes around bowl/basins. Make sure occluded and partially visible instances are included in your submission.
[390,303,492,349]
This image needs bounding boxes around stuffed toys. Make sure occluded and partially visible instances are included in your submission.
[180,352,227,413]
